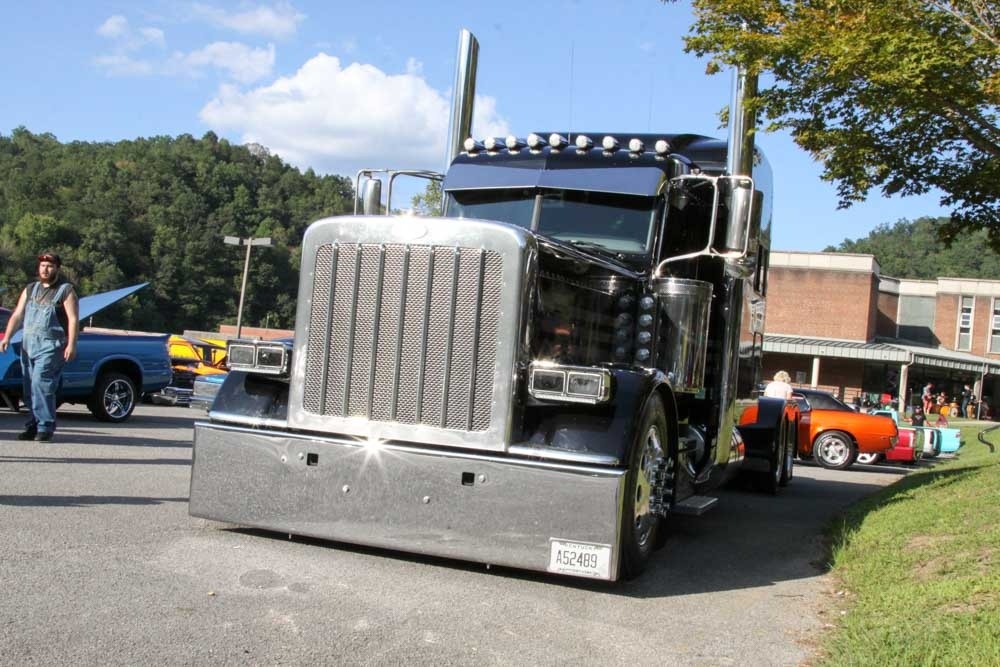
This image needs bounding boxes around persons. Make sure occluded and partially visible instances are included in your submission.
[904,406,931,427]
[0,252,79,443]
[936,391,946,407]
[764,371,793,401]
[961,384,972,418]
[921,382,935,415]
[852,392,872,407]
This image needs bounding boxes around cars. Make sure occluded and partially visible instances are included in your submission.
[735,380,899,470]
[140,332,228,412]
[0,281,175,423]
[846,401,960,465]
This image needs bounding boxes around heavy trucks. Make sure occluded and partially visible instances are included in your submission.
[188,23,800,584]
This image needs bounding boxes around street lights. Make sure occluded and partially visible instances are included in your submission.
[224,234,277,340]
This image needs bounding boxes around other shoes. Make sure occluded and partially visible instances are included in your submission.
[18,431,37,440]
[35,431,54,443]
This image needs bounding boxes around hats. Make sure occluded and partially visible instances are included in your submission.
[39,252,62,266]
